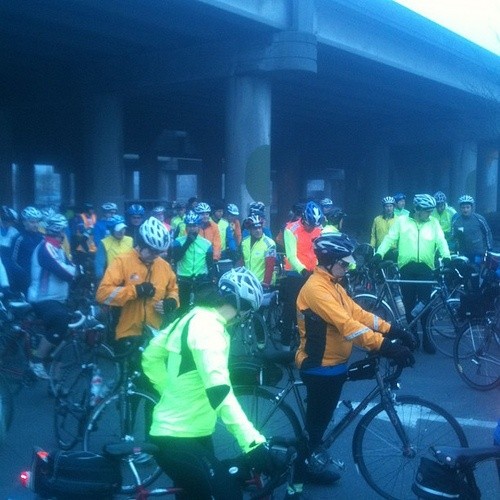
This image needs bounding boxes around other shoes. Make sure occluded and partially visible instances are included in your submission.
[423,337,435,353]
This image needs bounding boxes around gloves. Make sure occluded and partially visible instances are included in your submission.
[385,325,416,352]
[255,437,285,480]
[136,281,155,298]
[443,258,451,267]
[369,255,383,266]
[377,339,414,367]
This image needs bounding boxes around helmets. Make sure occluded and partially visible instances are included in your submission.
[0,193,476,235]
[138,217,170,252]
[312,233,354,263]
[216,266,265,314]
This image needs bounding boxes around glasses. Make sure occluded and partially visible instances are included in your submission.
[337,260,350,269]
[147,246,163,255]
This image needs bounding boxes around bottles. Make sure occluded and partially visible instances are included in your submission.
[90,369,102,407]
[96,381,116,404]
[393,293,406,317]
[321,399,352,439]
[411,297,430,317]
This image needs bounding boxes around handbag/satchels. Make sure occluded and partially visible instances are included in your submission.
[225,355,281,393]
[35,449,122,499]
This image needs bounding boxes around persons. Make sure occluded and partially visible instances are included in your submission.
[432,190,458,233]
[294,234,416,487]
[394,193,409,216]
[369,197,398,250]
[96,216,180,466]
[373,193,451,354]
[142,266,270,500]
[153,196,356,351]
[451,194,493,263]
[0,204,144,379]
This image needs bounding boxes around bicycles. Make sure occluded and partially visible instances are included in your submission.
[0,294,302,500]
[413,441,500,499]
[242,240,500,381]
[231,337,470,500]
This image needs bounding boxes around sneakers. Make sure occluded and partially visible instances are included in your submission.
[29,361,49,380]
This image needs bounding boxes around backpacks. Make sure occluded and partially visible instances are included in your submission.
[280,269,313,346]
